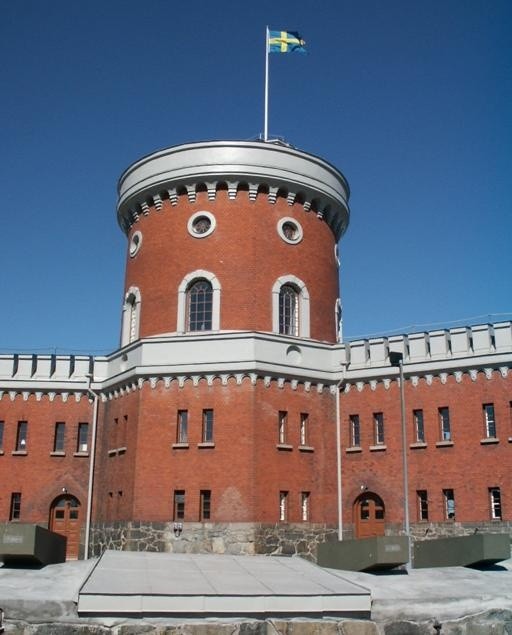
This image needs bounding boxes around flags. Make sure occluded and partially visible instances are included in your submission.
[269,29,305,55]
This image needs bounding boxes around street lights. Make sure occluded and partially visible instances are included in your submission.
[385,352,411,567]
